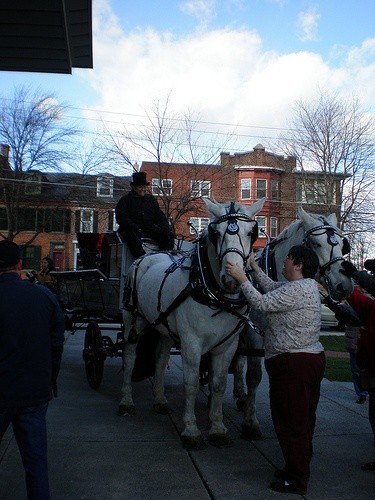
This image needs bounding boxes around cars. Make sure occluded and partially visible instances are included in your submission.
[320,302,340,332]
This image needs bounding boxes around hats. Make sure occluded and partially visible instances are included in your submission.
[130,172,151,186]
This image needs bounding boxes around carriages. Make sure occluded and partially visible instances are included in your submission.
[48,197,355,451]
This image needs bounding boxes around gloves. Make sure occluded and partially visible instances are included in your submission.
[339,260,357,277]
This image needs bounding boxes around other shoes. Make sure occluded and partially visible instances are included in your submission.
[356,395,366,404]
[268,479,307,495]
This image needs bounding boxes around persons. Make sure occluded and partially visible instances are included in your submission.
[115,171,175,261]
[225,245,327,494]
[0,240,65,500]
[319,259,375,471]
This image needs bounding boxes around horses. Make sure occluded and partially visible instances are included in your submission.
[232,205,354,441]
[116,196,269,452]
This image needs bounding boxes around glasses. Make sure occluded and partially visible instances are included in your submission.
[286,255,295,261]
